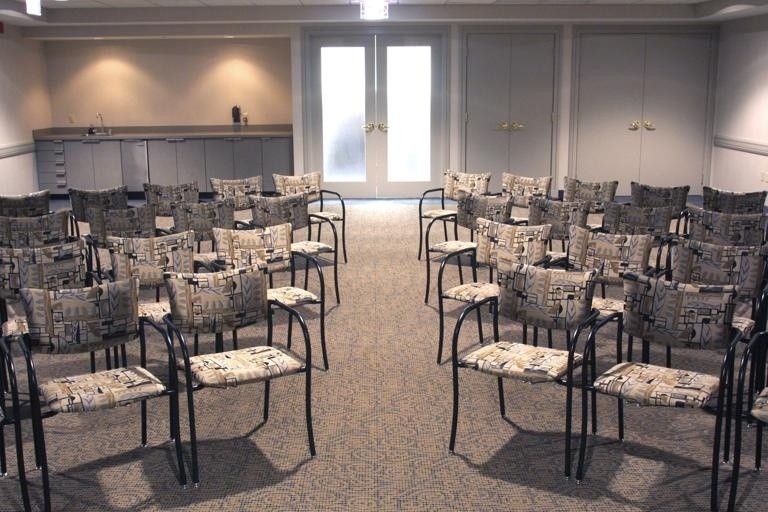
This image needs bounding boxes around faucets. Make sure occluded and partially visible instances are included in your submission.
[96,112,105,132]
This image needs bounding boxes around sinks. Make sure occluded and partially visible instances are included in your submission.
[82,133,113,137]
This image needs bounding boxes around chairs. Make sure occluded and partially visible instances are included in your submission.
[639,236,765,428]
[573,270,756,512]
[435,218,559,365]
[106,229,221,369]
[0,172,346,272]
[725,327,768,511]
[161,264,318,488]
[1,237,114,388]
[18,271,191,512]
[211,222,331,372]
[424,189,517,304]
[246,190,343,305]
[542,225,660,361]
[1,338,35,511]
[416,166,768,270]
[449,258,601,482]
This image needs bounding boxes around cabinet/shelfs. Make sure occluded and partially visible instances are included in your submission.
[35,124,294,197]
[460,23,562,202]
[566,20,717,198]
